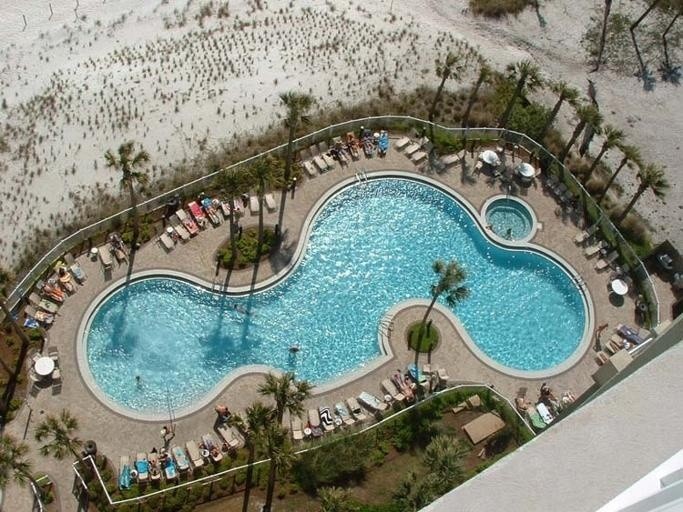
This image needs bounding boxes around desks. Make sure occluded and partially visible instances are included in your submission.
[334,418,343,427]
[303,427,311,436]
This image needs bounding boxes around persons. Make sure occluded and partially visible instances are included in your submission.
[377,129,388,153]
[33,266,79,328]
[163,424,172,434]
[198,442,229,457]
[515,398,532,413]
[165,454,172,464]
[129,470,138,479]
[214,404,228,417]
[147,459,160,476]
[348,133,360,159]
[176,196,222,236]
[393,368,413,400]
[226,203,241,212]
[335,138,350,162]
[359,127,375,150]
[110,234,131,257]
[540,383,558,403]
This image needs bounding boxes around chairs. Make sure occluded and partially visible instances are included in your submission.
[201,433,223,463]
[575,224,648,361]
[290,413,303,441]
[99,246,113,273]
[170,446,190,472]
[380,365,452,405]
[512,381,578,431]
[135,451,148,482]
[306,407,323,438]
[318,405,335,431]
[299,125,575,208]
[109,233,128,267]
[356,390,388,414]
[161,450,179,480]
[118,455,132,489]
[185,439,205,468]
[334,400,355,426]
[23,253,85,395]
[161,188,279,253]
[147,449,162,482]
[345,396,368,423]
